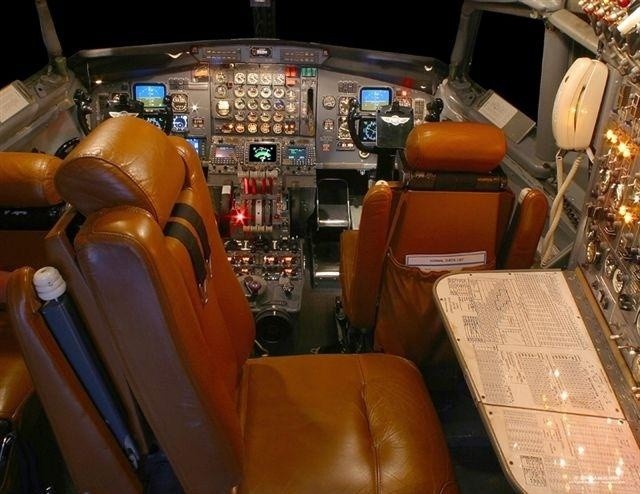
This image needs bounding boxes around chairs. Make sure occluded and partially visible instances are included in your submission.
[0,115,548,494]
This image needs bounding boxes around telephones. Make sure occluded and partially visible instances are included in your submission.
[551,57,609,152]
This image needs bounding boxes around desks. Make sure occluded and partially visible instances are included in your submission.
[431,268,640,494]
